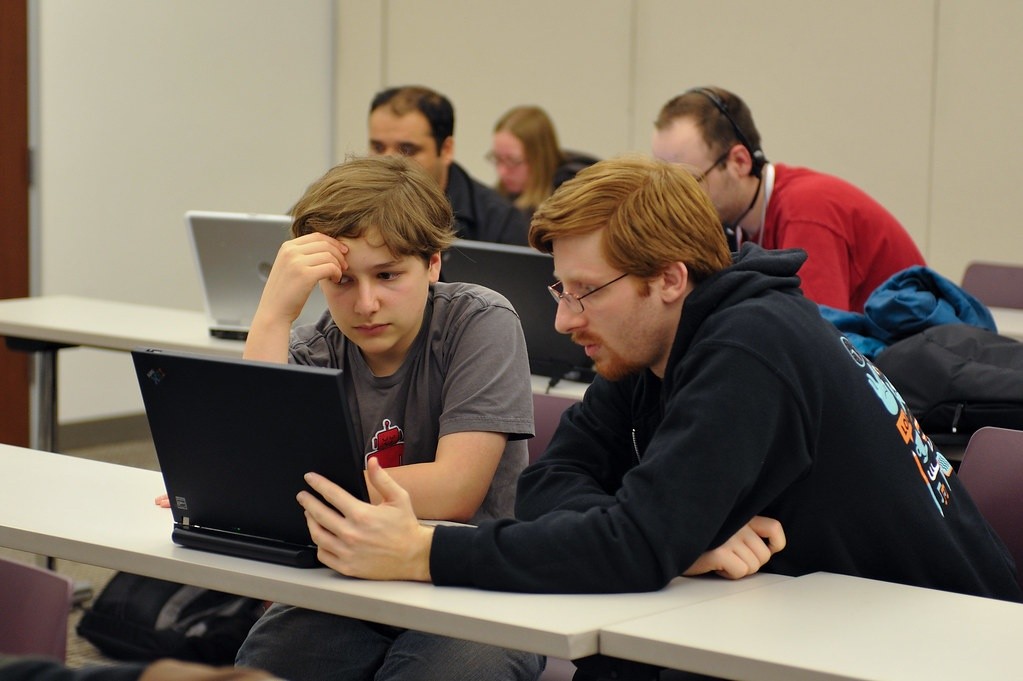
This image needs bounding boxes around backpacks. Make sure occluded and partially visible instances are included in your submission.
[874,325,1023,443]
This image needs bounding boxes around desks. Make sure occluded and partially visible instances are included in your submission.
[0,262,1023,680]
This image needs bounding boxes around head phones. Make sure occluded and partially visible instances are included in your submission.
[690,89,767,181]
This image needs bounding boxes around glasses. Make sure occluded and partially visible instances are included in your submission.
[548,273,630,313]
[486,151,524,167]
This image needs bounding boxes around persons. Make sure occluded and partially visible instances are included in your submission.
[294,163,1022,603]
[369,79,539,258]
[482,108,600,216]
[239,157,552,680]
[648,86,937,307]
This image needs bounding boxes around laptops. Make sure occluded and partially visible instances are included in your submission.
[188,212,591,380]
[130,345,373,568]
